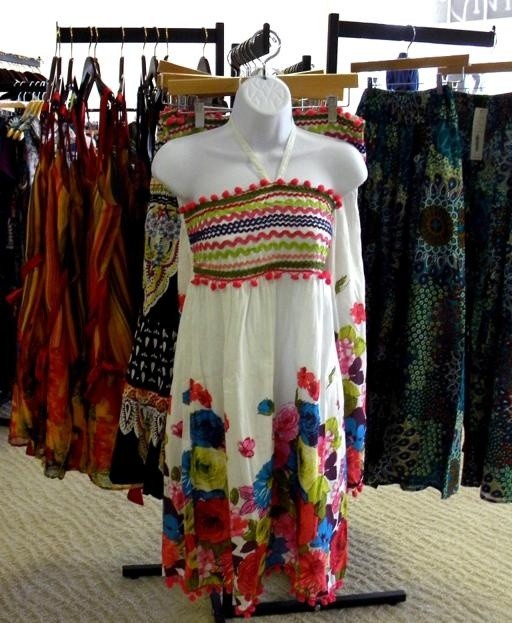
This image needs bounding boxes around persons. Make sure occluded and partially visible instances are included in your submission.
[109,75,370,619]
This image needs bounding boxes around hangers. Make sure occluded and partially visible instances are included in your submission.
[0,24,512,142]
